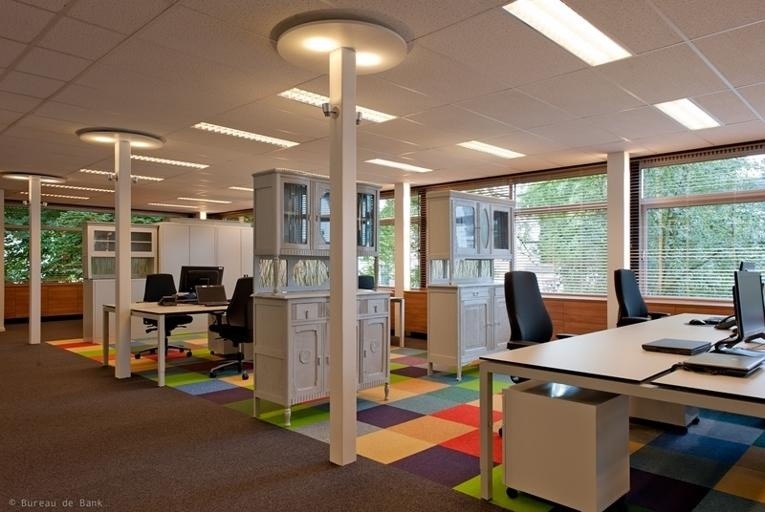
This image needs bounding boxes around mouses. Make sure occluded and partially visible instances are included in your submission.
[688,319,705,326]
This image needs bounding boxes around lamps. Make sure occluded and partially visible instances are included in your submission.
[355,112,363,125]
[322,103,340,119]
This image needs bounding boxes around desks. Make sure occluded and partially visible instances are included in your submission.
[102,301,229,388]
[478,313,765,512]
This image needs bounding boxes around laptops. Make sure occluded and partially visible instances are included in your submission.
[683,352,765,376]
[195,285,229,307]
[642,337,711,355]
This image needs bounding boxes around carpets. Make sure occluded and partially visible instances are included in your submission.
[45,331,765,511]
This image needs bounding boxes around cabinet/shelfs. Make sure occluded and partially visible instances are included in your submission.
[501,378,700,512]
[81,220,254,343]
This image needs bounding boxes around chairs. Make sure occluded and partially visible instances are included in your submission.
[499,271,579,437]
[614,269,671,328]
[134,273,253,381]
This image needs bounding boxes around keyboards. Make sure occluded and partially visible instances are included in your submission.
[704,316,734,324]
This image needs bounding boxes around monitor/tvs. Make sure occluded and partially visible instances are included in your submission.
[733,271,765,338]
[739,261,756,270]
[178,266,226,294]
